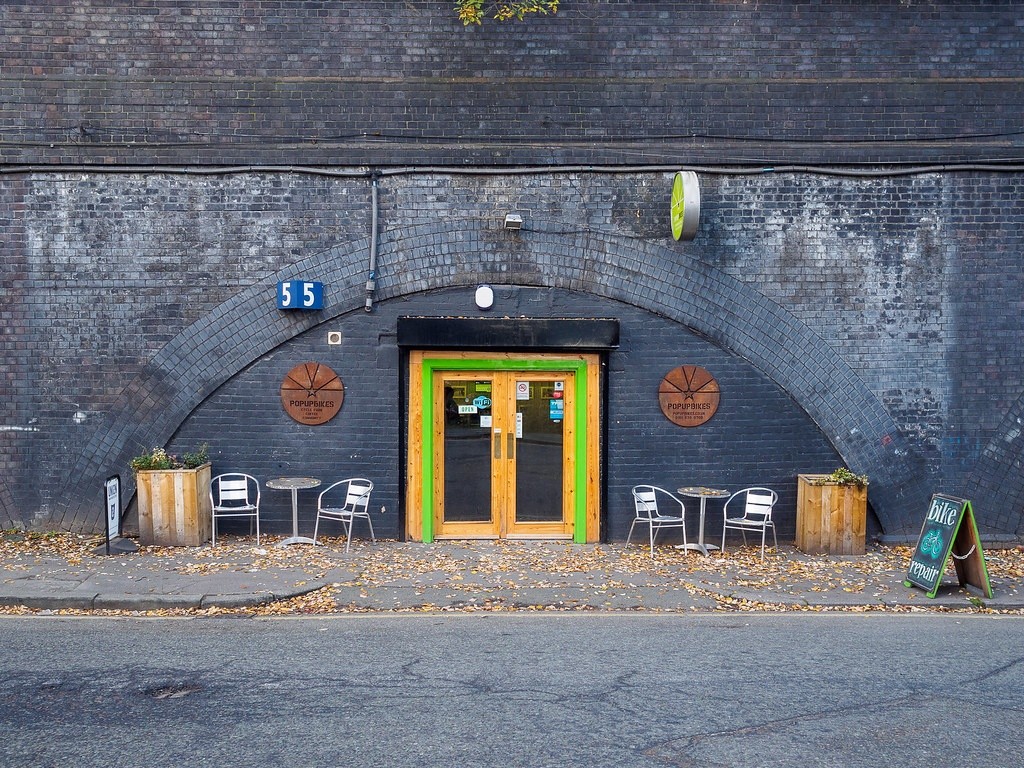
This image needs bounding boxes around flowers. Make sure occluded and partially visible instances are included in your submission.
[817,466,868,489]
[125,443,208,473]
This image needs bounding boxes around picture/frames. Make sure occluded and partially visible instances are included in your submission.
[452,386,467,399]
[529,387,534,399]
[541,387,555,400]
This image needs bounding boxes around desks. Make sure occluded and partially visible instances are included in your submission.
[676,488,731,558]
[265,478,322,549]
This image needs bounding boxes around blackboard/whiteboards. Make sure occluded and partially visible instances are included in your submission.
[905,496,964,593]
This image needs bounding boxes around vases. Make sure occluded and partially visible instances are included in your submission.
[794,475,868,554]
[136,463,213,546]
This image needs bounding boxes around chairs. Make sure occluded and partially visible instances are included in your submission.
[721,488,777,564]
[625,484,688,559]
[209,473,261,547]
[313,477,376,553]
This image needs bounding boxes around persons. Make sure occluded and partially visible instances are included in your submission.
[444,387,458,426]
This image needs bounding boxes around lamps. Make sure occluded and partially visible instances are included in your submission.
[502,211,525,230]
